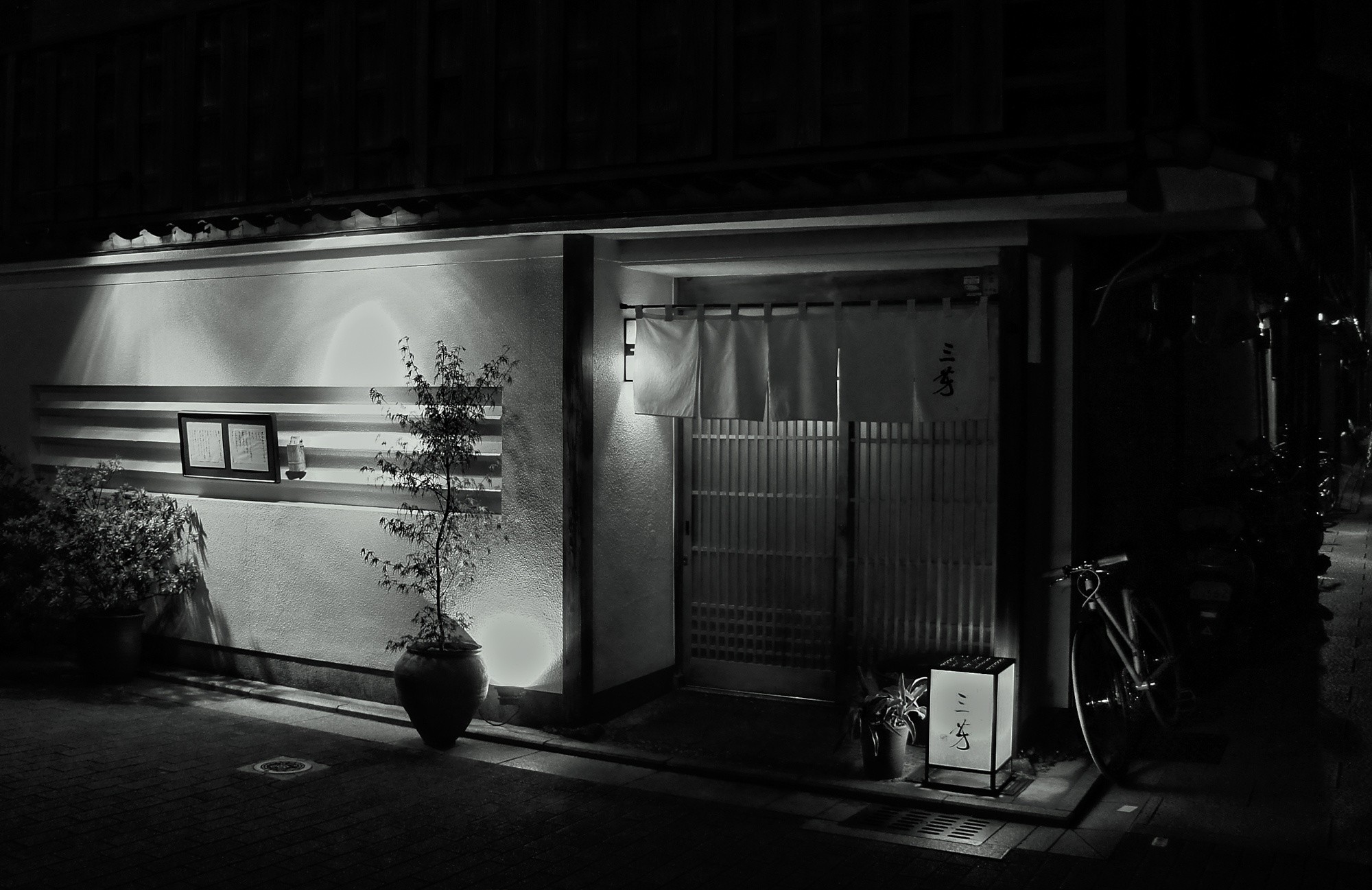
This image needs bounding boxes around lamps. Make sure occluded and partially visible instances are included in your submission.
[921,654,1015,797]
[624,318,636,382]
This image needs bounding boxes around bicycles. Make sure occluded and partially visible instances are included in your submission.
[1206,428,1340,551]
[1040,553,1185,787]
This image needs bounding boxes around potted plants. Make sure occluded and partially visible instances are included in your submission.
[360,336,523,751]
[1,455,202,683]
[845,673,927,780]
